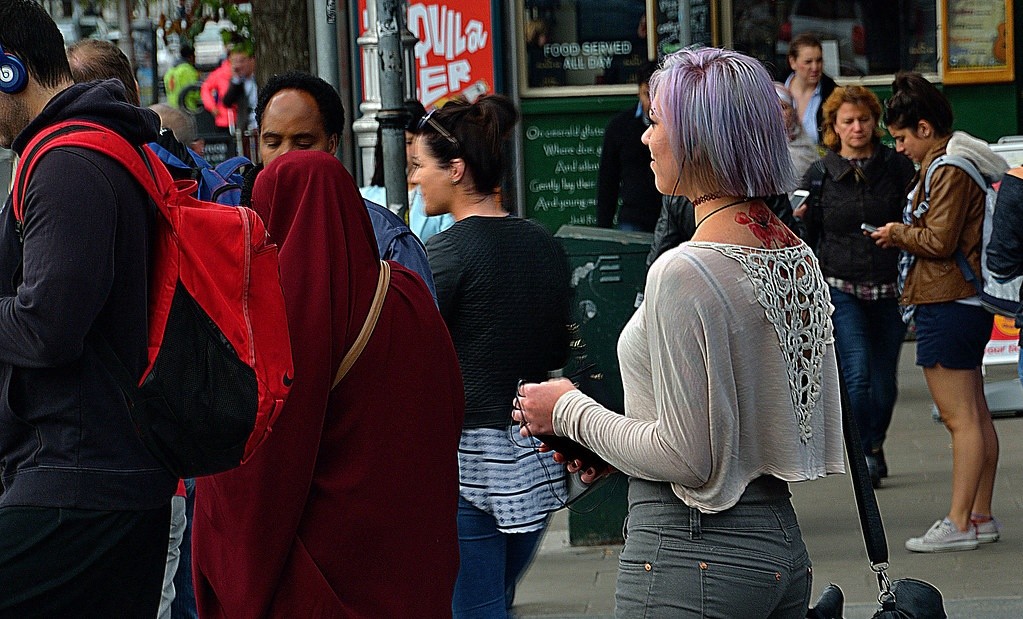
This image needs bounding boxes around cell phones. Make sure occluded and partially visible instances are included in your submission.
[861,223,877,233]
[790,189,810,210]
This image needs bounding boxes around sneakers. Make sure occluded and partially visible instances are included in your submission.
[971,514,1001,544]
[904,518,979,553]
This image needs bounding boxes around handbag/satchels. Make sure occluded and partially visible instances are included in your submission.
[871,577,948,618]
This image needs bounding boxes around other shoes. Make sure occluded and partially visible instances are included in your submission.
[865,448,888,488]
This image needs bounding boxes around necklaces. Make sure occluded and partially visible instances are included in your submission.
[696,197,761,227]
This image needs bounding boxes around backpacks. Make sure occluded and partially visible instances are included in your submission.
[925,154,1023,319]
[149,139,256,207]
[14,119,294,477]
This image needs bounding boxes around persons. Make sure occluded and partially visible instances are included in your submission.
[798,83,912,485]
[511,47,844,619]
[410,95,568,619]
[163,47,200,113]
[1,0,179,619]
[201,48,241,156]
[986,165,1023,382]
[224,45,259,157]
[191,150,466,619]
[864,73,1000,552]
[66,40,439,619]
[596,34,840,265]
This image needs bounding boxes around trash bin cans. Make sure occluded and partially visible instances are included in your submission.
[552,223,654,546]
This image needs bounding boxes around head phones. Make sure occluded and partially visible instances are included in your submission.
[0,46,29,95]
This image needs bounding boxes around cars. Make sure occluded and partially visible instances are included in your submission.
[776,1,868,78]
[129,18,230,78]
[54,18,119,46]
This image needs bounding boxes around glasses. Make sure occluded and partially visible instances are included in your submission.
[418,107,459,149]
